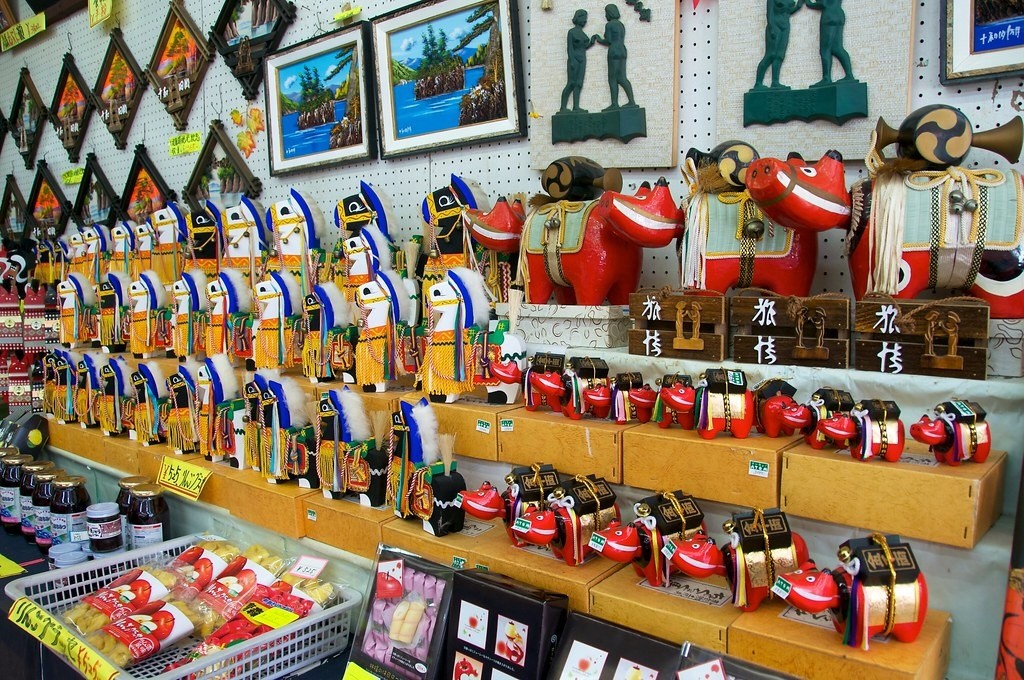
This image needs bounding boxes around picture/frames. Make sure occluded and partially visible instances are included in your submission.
[0,0,528,247]
[939,0,1024,86]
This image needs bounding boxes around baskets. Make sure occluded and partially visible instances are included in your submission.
[5,532,362,680]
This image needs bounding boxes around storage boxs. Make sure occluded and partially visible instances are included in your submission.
[339,543,461,680]
[622,421,805,508]
[434,567,571,680]
[382,511,506,569]
[589,563,742,654]
[227,469,323,539]
[318,378,416,441]
[65,423,127,465]
[103,435,155,475]
[44,415,96,452]
[495,293,990,381]
[468,532,629,616]
[780,438,1006,550]
[140,442,203,485]
[496,405,639,486]
[302,492,400,561]
[728,600,952,678]
[401,388,524,463]
[185,457,251,511]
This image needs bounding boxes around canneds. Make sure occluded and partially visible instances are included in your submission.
[0,446,170,600]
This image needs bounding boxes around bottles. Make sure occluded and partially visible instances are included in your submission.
[0,447,169,567]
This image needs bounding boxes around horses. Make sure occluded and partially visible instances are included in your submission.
[44,173,524,537]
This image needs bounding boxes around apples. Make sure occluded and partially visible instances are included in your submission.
[193,558,213,587]
[128,615,158,632]
[163,614,294,680]
[174,546,204,566]
[111,585,136,601]
[216,576,243,593]
[151,611,175,639]
[216,555,247,580]
[130,579,152,608]
[235,569,256,595]
[251,580,313,617]
